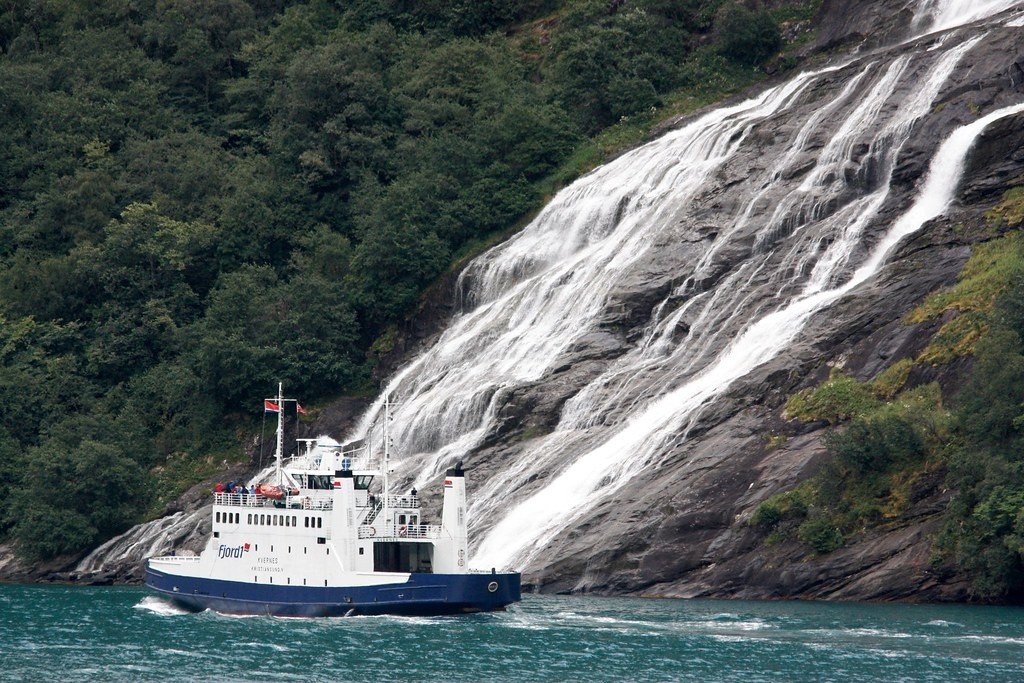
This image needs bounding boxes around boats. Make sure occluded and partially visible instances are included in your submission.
[142,383,524,620]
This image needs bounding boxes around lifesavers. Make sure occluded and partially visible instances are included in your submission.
[368,527,376,537]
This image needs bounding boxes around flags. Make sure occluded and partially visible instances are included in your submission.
[264,400,280,413]
[296,401,308,416]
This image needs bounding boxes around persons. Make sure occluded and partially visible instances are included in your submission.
[411,487,417,508]
[420,518,431,537]
[368,493,375,508]
[408,519,413,538]
[214,480,263,499]
